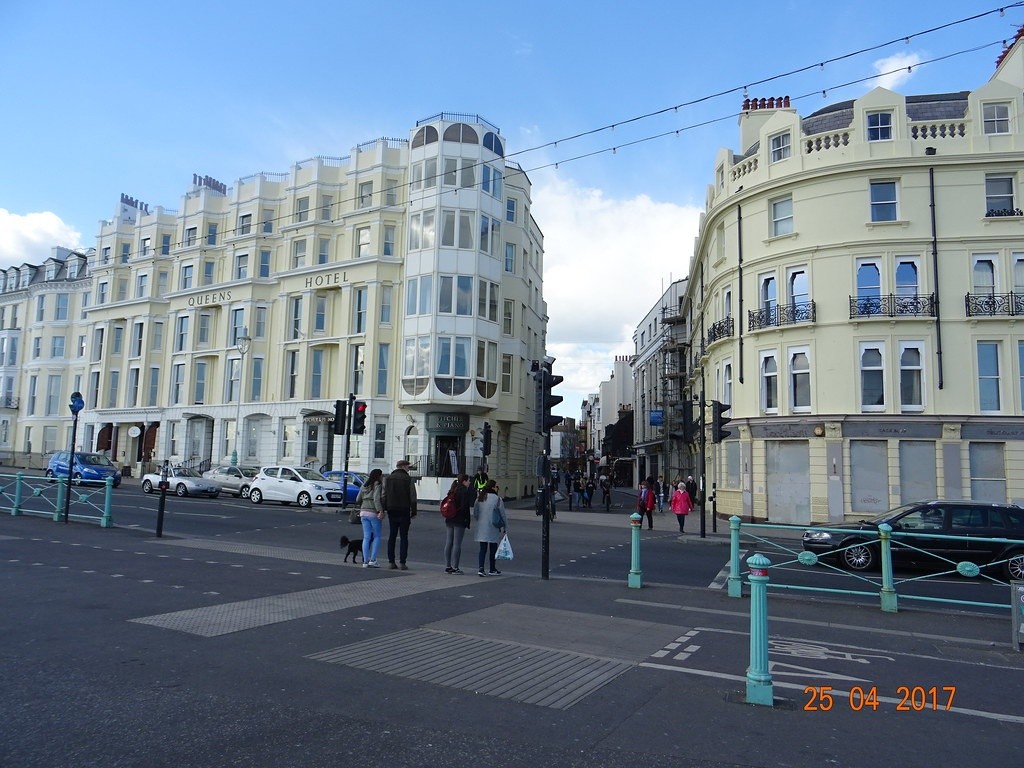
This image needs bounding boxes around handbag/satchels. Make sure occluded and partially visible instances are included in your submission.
[492,496,505,528]
[495,534,514,560]
[348,502,361,524]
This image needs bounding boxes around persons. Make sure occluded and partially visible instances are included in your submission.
[356,469,384,568]
[444,474,474,575]
[473,465,489,497]
[474,480,509,577]
[564,470,618,509]
[377,460,417,570]
[671,482,693,533]
[637,481,655,530]
[645,472,698,513]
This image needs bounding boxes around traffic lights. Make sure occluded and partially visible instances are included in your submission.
[332,400,346,435]
[353,402,367,434]
[668,400,693,442]
[713,400,732,442]
[480,425,492,455]
[541,367,564,437]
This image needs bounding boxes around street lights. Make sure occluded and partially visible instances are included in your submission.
[230,326,253,465]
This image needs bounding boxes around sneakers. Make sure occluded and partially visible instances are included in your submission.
[478,567,486,577]
[489,569,503,576]
[444,567,464,575]
[362,558,382,568]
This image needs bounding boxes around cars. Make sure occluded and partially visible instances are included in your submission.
[45,451,122,488]
[323,471,386,510]
[140,466,222,498]
[200,465,260,499]
[248,466,344,507]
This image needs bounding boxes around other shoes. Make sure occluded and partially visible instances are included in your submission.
[399,563,408,570]
[389,563,398,569]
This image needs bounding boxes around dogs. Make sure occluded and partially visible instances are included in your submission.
[339,535,364,563]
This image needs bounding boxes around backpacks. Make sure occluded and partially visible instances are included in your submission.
[440,485,468,519]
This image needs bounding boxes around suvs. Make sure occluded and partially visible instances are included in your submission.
[801,496,1024,579]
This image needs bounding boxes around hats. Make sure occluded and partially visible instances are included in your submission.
[396,460,410,466]
[688,476,693,480]
